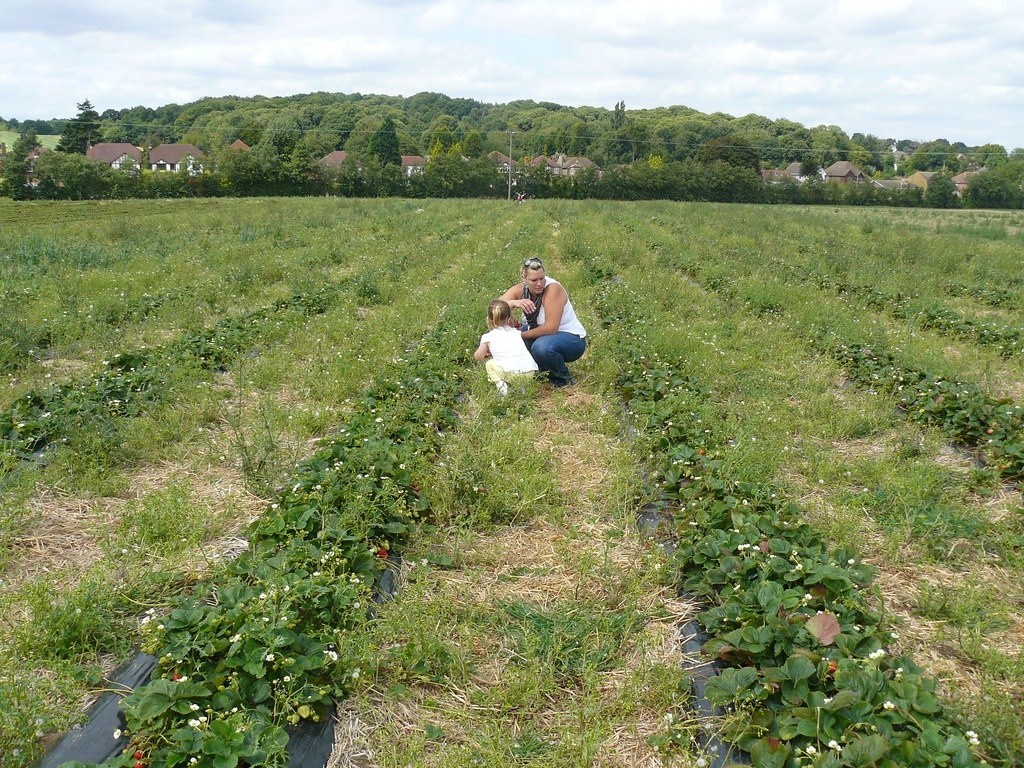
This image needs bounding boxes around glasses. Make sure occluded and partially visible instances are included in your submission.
[522,257,542,271]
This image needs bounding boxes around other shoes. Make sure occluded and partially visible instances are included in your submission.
[553,379,575,387]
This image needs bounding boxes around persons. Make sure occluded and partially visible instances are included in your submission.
[498,258,587,387]
[474,300,538,395]
